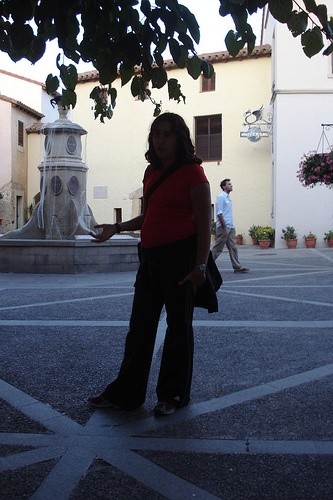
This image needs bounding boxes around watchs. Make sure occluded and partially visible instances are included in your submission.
[196,264,207,271]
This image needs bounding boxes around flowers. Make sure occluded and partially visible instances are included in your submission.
[297,143,333,190]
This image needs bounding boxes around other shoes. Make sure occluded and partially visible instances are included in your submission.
[154,401,175,414]
[233,267,250,274]
[87,393,118,407]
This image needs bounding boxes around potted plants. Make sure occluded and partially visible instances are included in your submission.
[248,224,275,249]
[323,229,333,248]
[280,225,298,249]
[303,231,316,248]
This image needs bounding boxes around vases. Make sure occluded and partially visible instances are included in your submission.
[236,234,243,246]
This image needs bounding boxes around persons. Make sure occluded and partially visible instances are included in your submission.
[87,113,212,415]
[212,179,249,272]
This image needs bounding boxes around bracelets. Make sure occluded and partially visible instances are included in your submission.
[115,223,121,234]
